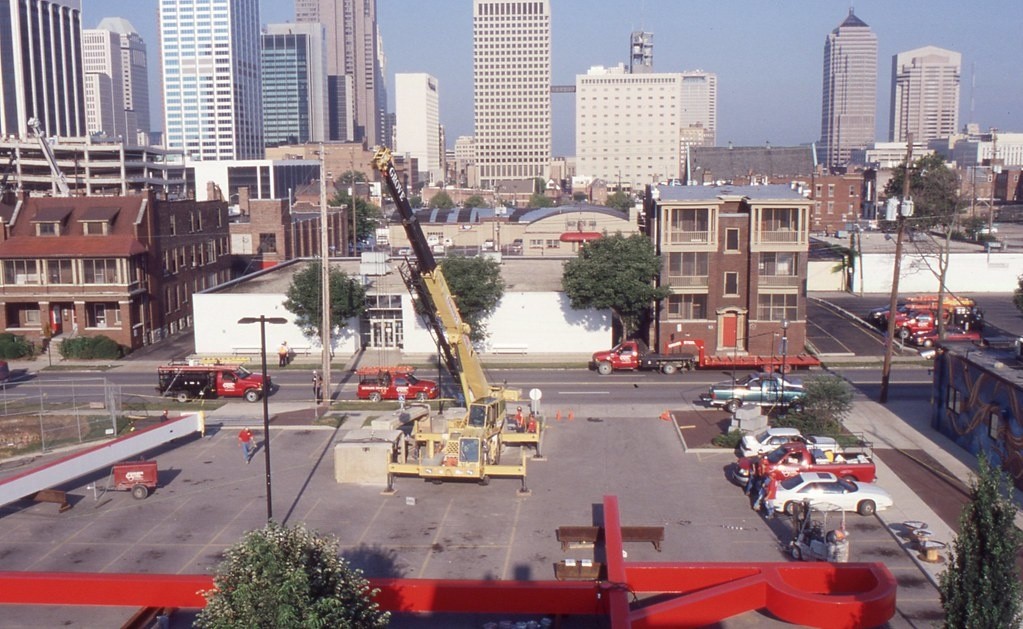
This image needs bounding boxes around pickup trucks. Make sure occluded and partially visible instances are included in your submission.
[354,365,440,403]
[735,442,877,485]
[593,338,693,375]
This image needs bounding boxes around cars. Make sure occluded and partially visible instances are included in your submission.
[349,235,522,257]
[739,428,840,459]
[708,372,812,415]
[868,294,986,348]
[764,470,892,517]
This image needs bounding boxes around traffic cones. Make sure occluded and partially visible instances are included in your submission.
[555,409,564,421]
[567,408,575,421]
[660,413,672,422]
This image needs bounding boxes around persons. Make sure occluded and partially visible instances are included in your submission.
[747,449,778,520]
[312,370,323,405]
[282,341,290,365]
[277,342,287,367]
[515,406,524,433]
[526,414,536,449]
[237,427,257,465]
[471,407,484,426]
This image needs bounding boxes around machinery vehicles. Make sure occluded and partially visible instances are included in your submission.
[366,147,546,495]
[781,503,849,565]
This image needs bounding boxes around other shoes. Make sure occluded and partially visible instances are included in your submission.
[279,365,285,367]
[317,401,320,404]
[746,492,751,496]
[287,362,289,364]
[765,515,774,519]
[246,461,249,464]
[754,505,762,510]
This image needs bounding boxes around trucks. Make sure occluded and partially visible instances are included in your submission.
[156,356,273,402]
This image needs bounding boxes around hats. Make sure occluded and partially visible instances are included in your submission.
[763,455,768,459]
[312,371,317,373]
[281,341,286,345]
[758,449,763,453]
[770,472,776,477]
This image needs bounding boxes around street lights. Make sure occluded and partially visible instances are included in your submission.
[236,314,289,522]
[779,318,790,418]
